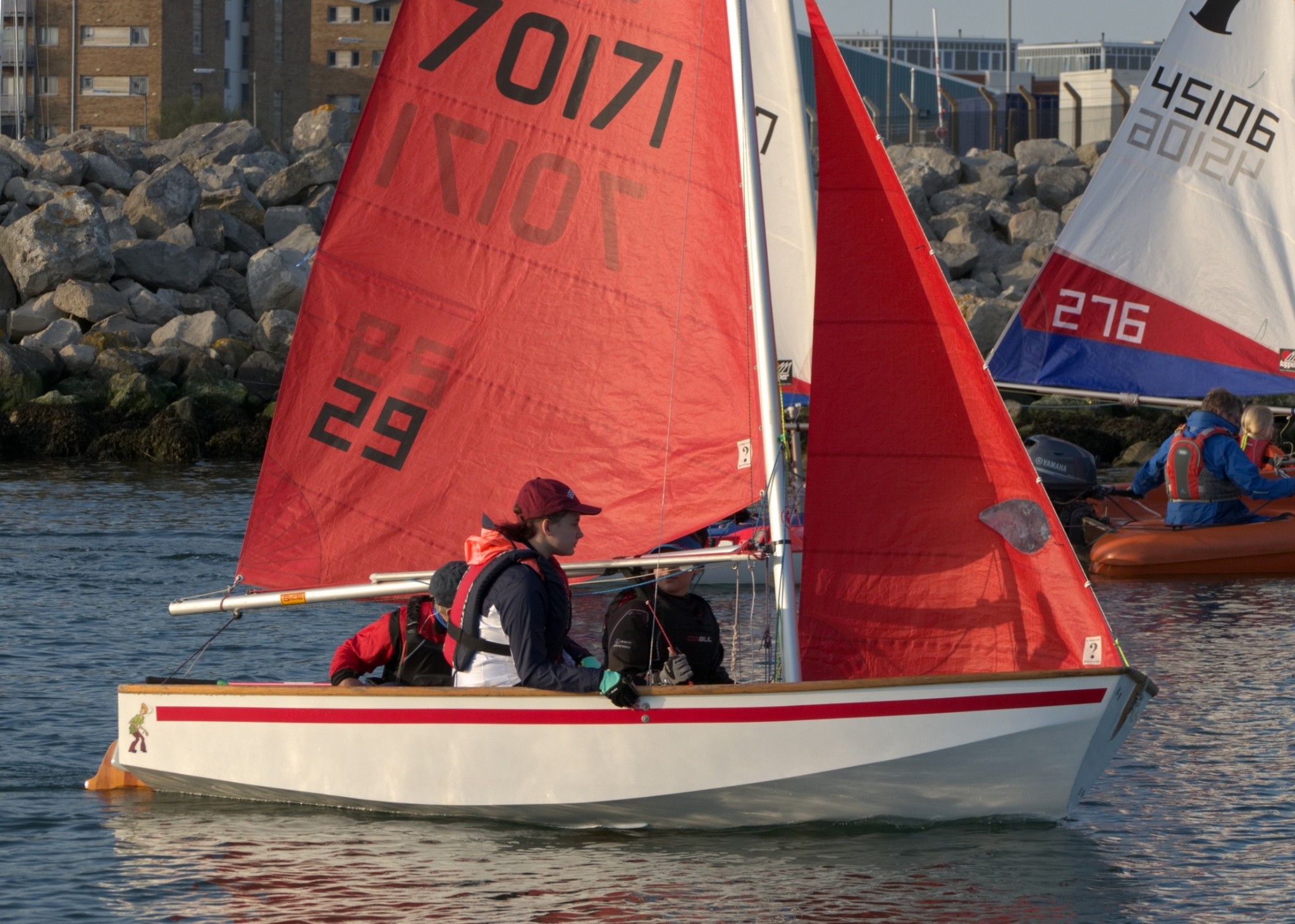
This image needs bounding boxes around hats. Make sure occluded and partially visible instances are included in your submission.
[649,535,703,554]
[429,561,469,599]
[516,477,602,521]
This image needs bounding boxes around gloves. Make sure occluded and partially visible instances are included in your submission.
[653,654,693,686]
[601,669,639,707]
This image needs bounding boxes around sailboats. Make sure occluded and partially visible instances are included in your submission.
[108,1,1162,835]
[983,0,1295,579]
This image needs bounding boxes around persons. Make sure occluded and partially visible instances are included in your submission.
[329,561,469,688]
[1125,387,1295,525]
[602,536,745,684]
[443,476,640,708]
[1234,406,1292,471]
